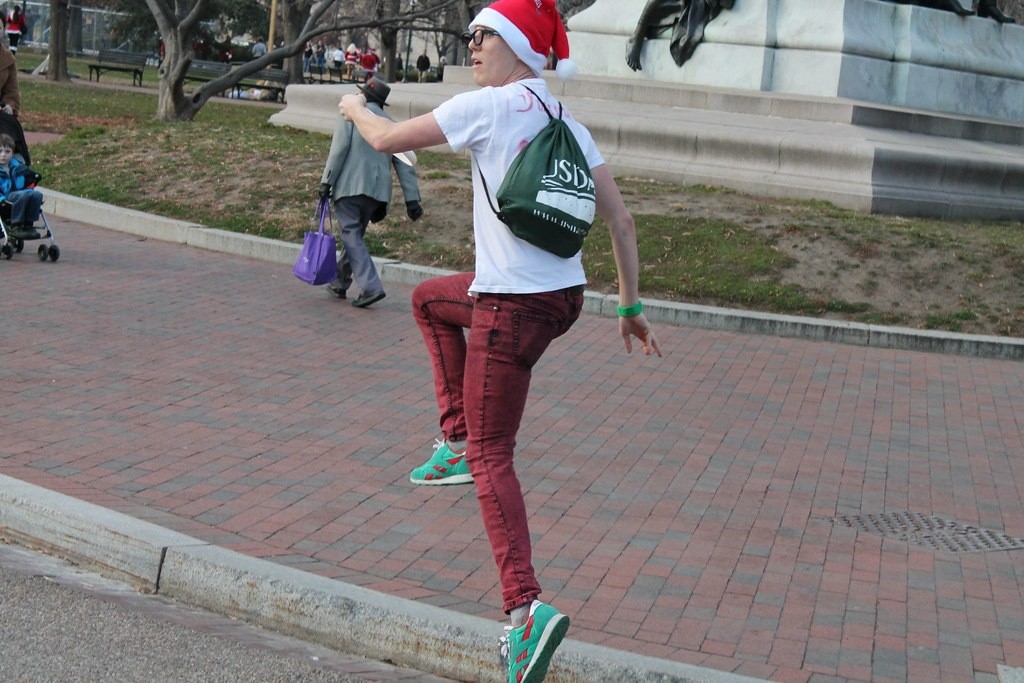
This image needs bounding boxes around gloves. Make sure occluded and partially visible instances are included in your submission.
[318,183,331,203]
[405,200,423,221]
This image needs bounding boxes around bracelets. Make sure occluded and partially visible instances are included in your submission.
[616,300,644,317]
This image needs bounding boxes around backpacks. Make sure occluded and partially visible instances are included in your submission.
[477,82,597,259]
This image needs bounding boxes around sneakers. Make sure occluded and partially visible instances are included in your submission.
[410,438,474,486]
[498,600,570,683]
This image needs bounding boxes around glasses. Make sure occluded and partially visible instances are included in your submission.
[460,29,500,48]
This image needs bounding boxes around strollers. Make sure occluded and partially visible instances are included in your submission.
[0,108,61,262]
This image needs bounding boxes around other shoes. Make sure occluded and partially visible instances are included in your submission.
[352,291,386,307]
[11,226,27,239]
[326,285,346,298]
[26,226,36,238]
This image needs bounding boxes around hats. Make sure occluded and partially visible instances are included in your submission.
[469,0,581,81]
[356,76,392,106]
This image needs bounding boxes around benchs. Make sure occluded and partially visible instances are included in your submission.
[183,57,231,95]
[308,64,342,81]
[231,67,290,103]
[86,48,148,87]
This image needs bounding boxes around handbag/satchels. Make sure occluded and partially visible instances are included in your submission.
[20,22,27,34]
[295,196,338,286]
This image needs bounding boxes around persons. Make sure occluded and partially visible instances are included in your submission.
[0,134,44,239]
[315,40,326,75]
[319,77,423,307]
[416,49,431,83]
[251,37,266,59]
[396,52,403,73]
[6,5,27,56]
[302,42,313,74]
[436,56,448,83]
[340,0,662,683]
[0,10,21,117]
[331,43,380,82]
